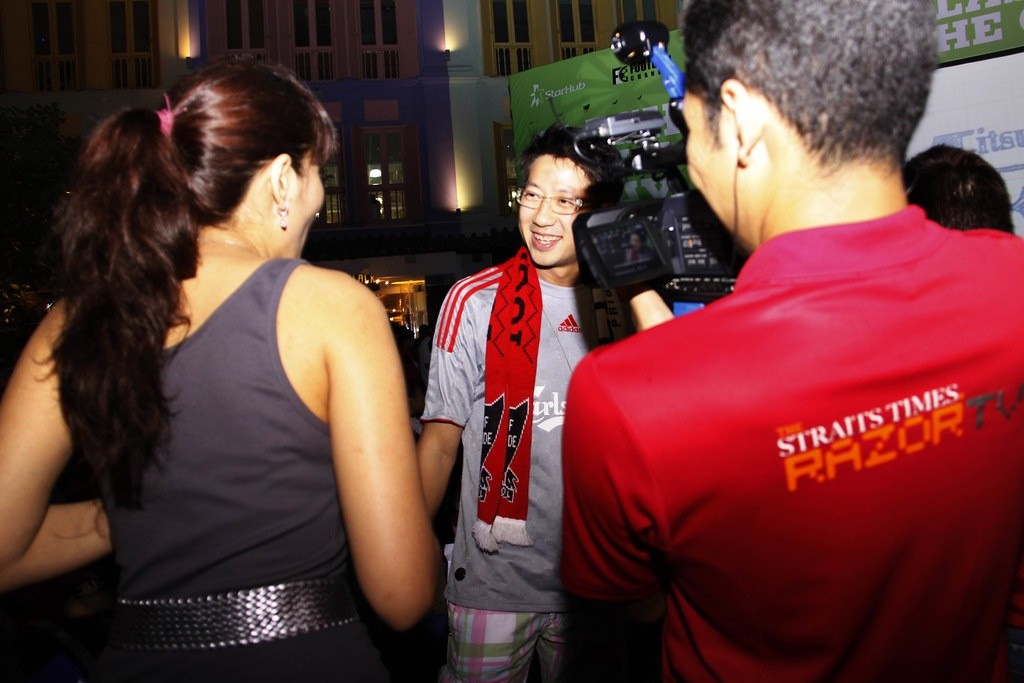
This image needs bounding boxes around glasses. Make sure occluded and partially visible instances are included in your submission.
[516,188,585,215]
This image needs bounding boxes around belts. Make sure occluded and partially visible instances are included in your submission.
[112,581,367,649]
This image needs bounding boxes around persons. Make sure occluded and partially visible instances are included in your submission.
[621,233,653,265]
[901,144,1014,235]
[560,0,1024,683]
[414,125,629,683]
[0,63,444,682]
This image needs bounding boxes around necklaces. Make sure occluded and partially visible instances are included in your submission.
[193,236,249,250]
